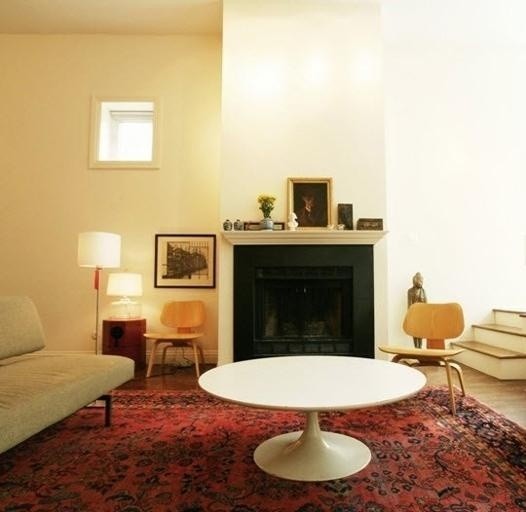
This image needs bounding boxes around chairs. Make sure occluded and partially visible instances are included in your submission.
[140,298,209,383]
[378,301,468,417]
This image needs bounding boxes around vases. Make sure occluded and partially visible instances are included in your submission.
[233,218,244,230]
[260,216,274,230]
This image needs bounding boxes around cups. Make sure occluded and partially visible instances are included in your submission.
[327,224,335,230]
[337,224,345,230]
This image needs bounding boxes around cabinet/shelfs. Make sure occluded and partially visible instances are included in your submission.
[101,317,147,372]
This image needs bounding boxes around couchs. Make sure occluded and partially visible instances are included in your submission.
[0,291,136,457]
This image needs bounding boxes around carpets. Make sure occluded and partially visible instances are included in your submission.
[1,385,525,512]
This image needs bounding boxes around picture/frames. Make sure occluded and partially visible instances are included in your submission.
[151,231,217,291]
[285,175,335,231]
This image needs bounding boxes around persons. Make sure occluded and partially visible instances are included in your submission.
[407,272,427,349]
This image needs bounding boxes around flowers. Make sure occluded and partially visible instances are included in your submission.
[255,192,275,217]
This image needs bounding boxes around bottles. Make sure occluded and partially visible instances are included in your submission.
[234,219,243,230]
[287,211,299,231]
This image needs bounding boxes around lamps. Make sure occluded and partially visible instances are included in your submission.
[76,229,122,408]
[104,270,144,321]
